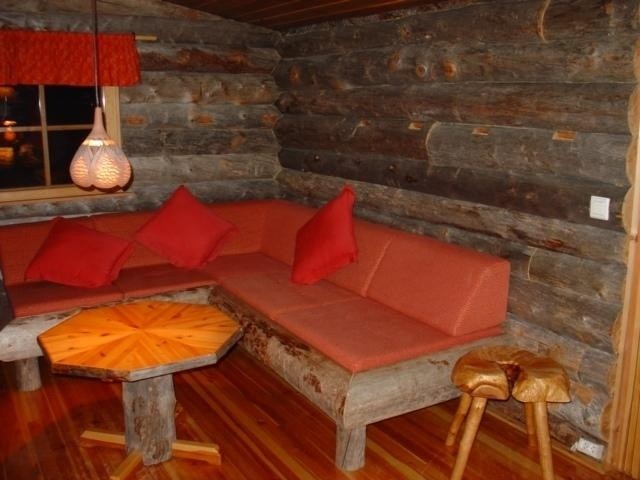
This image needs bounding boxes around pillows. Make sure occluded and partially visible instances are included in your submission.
[24,181,238,291]
[289,184,361,287]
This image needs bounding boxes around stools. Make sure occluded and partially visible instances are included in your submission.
[444,344,573,479]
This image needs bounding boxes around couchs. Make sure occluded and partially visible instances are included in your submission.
[1,197,513,475]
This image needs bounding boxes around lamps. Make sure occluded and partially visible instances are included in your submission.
[67,1,134,192]
